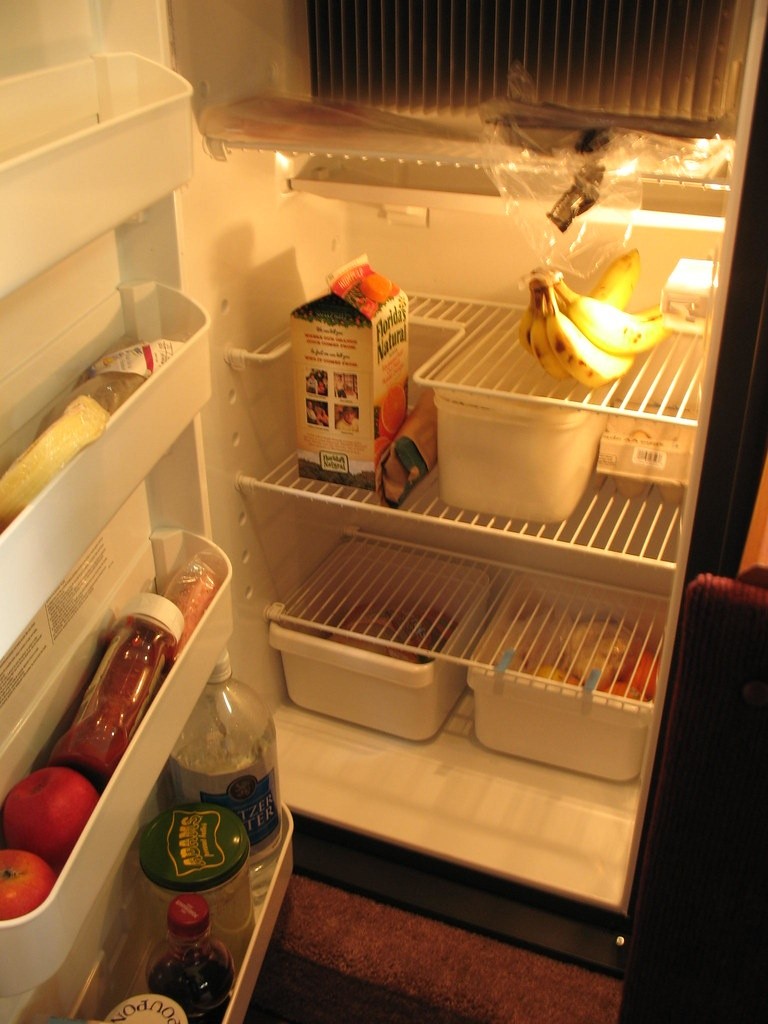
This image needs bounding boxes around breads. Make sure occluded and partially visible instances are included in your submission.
[0,394,111,536]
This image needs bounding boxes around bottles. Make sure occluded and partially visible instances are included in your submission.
[169,652,283,897]
[146,893,234,1024]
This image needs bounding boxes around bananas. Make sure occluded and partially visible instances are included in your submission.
[518,246,670,387]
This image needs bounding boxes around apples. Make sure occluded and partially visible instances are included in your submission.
[0,849,59,922]
[0,766,100,873]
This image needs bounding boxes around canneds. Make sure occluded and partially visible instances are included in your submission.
[137,802,256,974]
